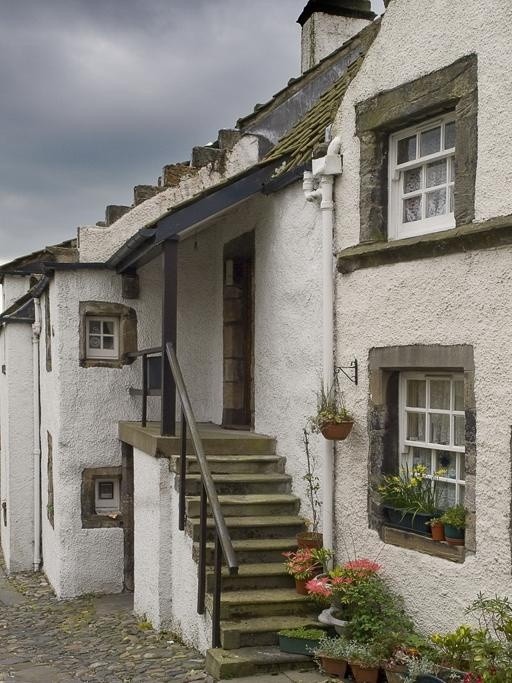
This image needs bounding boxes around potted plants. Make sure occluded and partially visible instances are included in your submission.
[296,427,323,550]
[424,518,444,541]
[276,625,326,655]
[440,504,469,539]
[304,407,357,440]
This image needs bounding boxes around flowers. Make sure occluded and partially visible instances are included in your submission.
[281,547,512,683]
[363,456,449,528]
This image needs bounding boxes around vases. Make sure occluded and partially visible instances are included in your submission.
[381,503,442,538]
